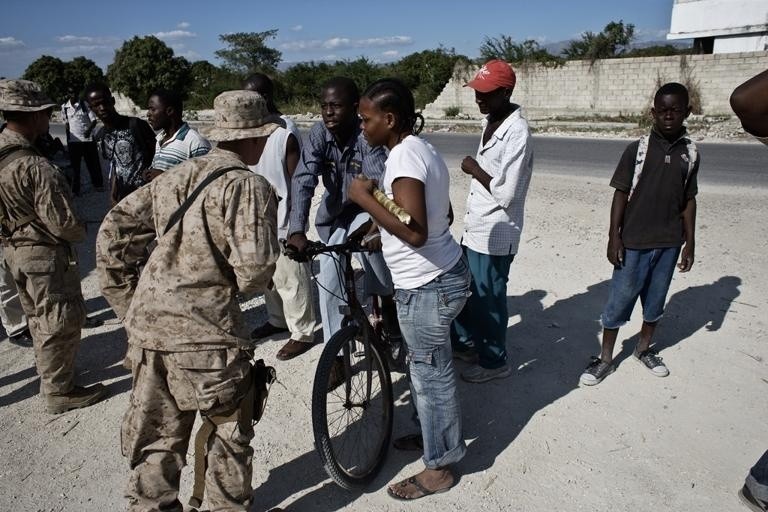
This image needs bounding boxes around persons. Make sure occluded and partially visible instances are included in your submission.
[96,89,288,511]
[729,68,768,512]
[1,79,212,414]
[579,81,701,387]
[452,59,535,384]
[284,75,408,394]
[240,72,318,361]
[347,77,473,501]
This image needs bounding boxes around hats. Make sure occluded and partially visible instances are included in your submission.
[463,59,516,93]
[0,80,58,112]
[198,89,286,142]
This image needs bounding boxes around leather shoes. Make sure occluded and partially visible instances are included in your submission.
[9,329,33,347]
[327,357,346,393]
[85,318,104,328]
[276,339,313,360]
[251,322,287,339]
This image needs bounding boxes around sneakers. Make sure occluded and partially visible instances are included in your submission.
[461,359,511,383]
[46,384,105,415]
[632,342,669,377]
[738,484,768,512]
[580,356,616,386]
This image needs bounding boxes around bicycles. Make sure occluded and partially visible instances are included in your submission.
[277,224,393,491]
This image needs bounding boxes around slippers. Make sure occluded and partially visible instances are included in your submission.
[393,434,423,450]
[387,476,450,499]
